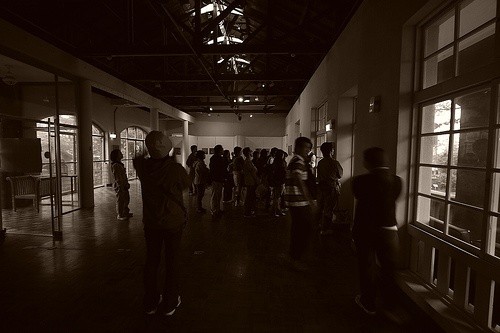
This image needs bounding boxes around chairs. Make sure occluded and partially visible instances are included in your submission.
[8,175,39,214]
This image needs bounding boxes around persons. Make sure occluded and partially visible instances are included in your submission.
[285,136,316,263]
[135,130,194,305]
[317,142,343,226]
[110,149,133,221]
[186,145,318,219]
[351,147,402,315]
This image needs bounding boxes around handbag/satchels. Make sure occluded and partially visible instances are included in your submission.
[306,169,317,199]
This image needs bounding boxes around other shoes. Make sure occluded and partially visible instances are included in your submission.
[278,212,286,215]
[247,210,256,215]
[292,261,307,272]
[117,215,127,220]
[273,214,279,217]
[355,294,376,315]
[127,213,133,216]
[165,296,182,315]
[145,294,163,314]
[197,209,207,213]
[223,201,231,204]
[281,208,288,212]
[320,229,334,235]
[243,215,253,219]
[235,204,244,207]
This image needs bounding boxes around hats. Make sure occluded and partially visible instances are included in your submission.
[145,131,172,160]
[243,147,252,154]
